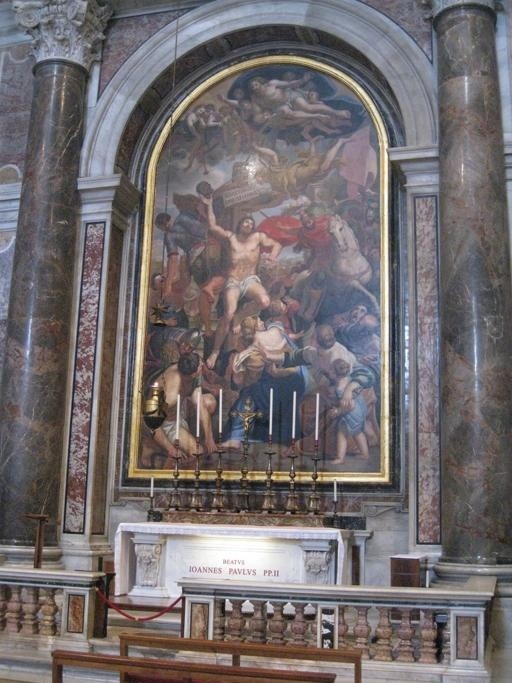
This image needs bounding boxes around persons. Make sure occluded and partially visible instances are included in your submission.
[146,69,378,468]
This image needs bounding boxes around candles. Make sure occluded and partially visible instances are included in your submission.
[174,383,223,439]
[268,386,321,440]
[333,477,338,502]
[149,474,154,499]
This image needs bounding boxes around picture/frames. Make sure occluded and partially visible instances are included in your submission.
[121,50,400,491]
